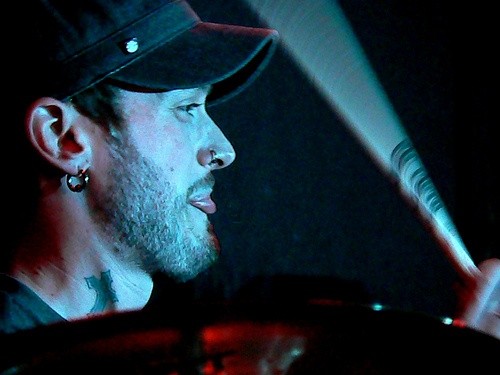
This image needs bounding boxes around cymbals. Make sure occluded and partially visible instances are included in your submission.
[0,301,500,375]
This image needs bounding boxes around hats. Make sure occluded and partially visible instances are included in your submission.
[1,0,279,106]
